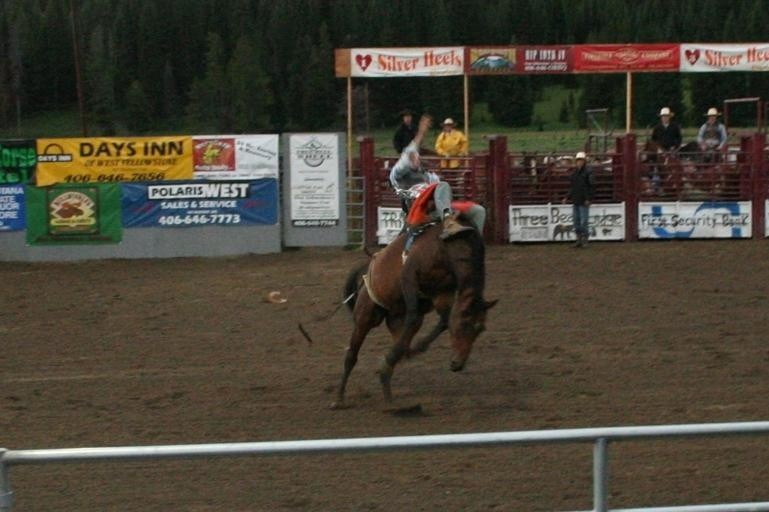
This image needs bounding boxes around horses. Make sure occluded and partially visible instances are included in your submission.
[329,213,500,409]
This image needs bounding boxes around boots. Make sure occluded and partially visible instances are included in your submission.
[570,236,590,249]
[439,214,474,241]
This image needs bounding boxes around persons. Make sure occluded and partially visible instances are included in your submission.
[561,148,595,250]
[393,109,420,152]
[388,113,487,241]
[698,104,728,179]
[649,106,681,192]
[431,116,467,168]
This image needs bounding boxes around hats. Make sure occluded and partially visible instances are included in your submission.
[657,106,723,116]
[399,109,457,126]
[543,152,590,165]
